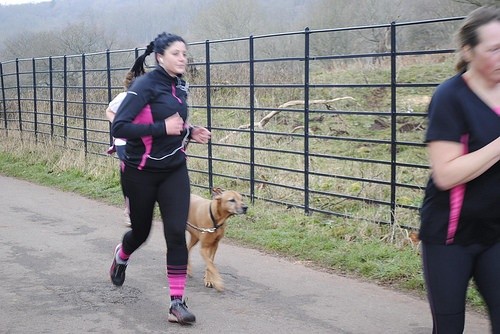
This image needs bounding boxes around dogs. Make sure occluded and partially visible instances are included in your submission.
[185,187,248,292]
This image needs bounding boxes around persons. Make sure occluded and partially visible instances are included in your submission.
[418,6,499,334]
[109,69,146,228]
[109,33,210,325]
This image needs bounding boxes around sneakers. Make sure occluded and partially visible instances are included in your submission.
[110,243,128,286]
[167,299,196,324]
[124,213,131,227]
